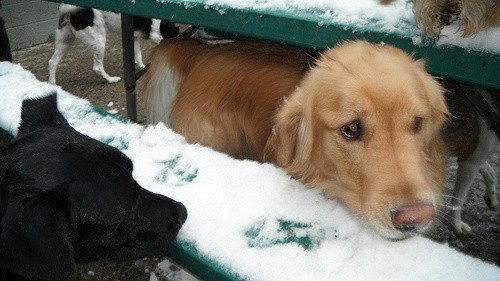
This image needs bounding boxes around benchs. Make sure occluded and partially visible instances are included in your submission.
[0,61,500,281]
[37,0,500,128]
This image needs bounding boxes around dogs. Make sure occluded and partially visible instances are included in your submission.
[1,89,189,280]
[140,37,450,242]
[376,0,500,40]
[46,3,179,86]
